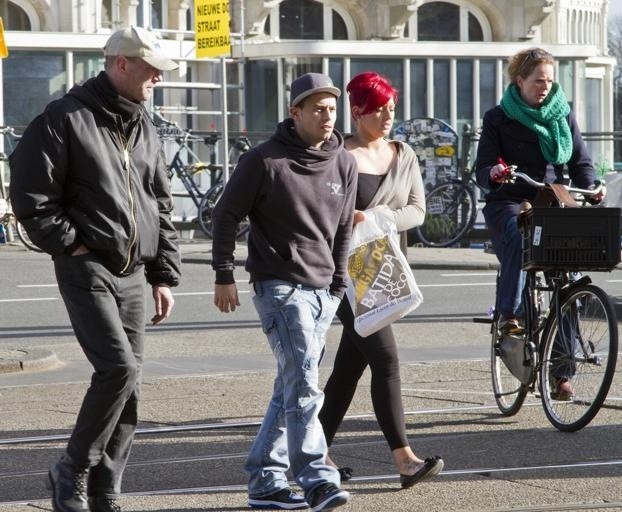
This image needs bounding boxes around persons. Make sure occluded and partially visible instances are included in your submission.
[211,73,359,512]
[8,26,182,512]
[318,72,442,489]
[475,49,607,398]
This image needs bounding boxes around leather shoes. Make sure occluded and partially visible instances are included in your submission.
[338,465,352,481]
[399,455,444,488]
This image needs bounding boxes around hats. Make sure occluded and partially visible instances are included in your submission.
[104,26,181,73]
[290,73,342,109]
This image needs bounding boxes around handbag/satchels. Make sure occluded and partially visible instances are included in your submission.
[345,205,423,338]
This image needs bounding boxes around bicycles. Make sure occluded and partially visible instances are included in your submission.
[0,124,47,252]
[474,165,622,431]
[153,126,253,241]
[415,129,490,247]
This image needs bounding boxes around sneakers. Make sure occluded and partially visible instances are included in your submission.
[93,498,117,510]
[552,379,573,400]
[48,456,90,512]
[500,315,528,337]
[303,478,356,511]
[246,486,310,511]
